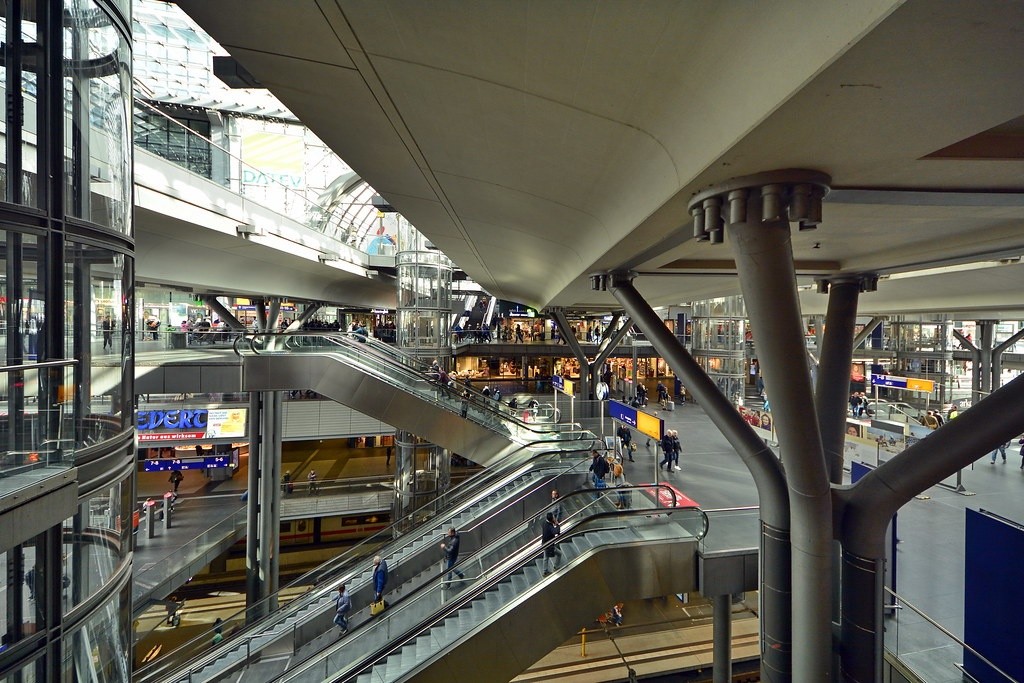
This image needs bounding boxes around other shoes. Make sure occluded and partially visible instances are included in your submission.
[543,572,551,577]
[460,573,465,578]
[660,463,663,470]
[668,469,674,472]
[441,586,451,589]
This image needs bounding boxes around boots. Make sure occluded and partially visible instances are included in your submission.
[614,500,624,509]
[629,456,634,462]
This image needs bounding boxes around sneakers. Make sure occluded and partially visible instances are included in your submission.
[675,466,681,470]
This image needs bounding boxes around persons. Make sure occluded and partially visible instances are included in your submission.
[807,325,826,345]
[386,447,391,465]
[429,296,685,421]
[282,469,318,497]
[290,389,319,399]
[102,316,116,350]
[916,329,973,375]
[372,556,389,608]
[451,453,476,469]
[27,568,36,600]
[25,316,45,334]
[589,412,686,509]
[332,584,352,635]
[744,330,771,413]
[165,597,177,624]
[845,424,896,447]
[210,618,240,646]
[919,405,1024,469]
[595,603,623,628]
[441,526,465,590]
[144,313,396,343]
[741,407,771,431]
[542,489,562,577]
[142,445,205,521]
[849,392,872,420]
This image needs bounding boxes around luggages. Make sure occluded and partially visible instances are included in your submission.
[667,396,674,410]
[173,610,180,625]
[624,482,632,508]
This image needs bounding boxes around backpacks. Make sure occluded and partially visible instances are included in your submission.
[597,456,609,473]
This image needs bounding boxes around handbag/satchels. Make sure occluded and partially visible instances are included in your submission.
[533,407,538,413]
[595,476,607,488]
[371,596,385,615]
[632,443,637,452]
